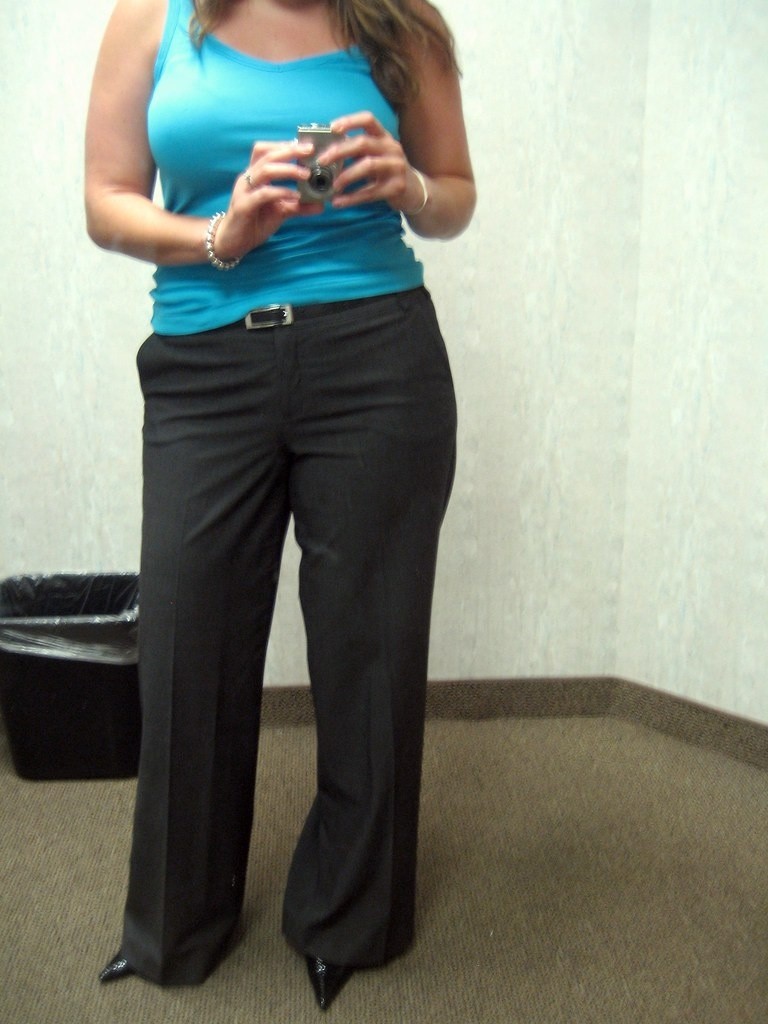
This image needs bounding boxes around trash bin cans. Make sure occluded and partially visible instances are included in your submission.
[0,572,141,781]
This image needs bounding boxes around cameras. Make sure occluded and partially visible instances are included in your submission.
[296,122,346,203]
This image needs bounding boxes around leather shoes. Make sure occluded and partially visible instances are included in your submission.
[100,950,134,981]
[307,957,354,1011]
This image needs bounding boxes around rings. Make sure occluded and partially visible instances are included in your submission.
[243,171,256,187]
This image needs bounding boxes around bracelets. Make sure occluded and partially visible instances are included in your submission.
[400,166,428,215]
[205,210,244,271]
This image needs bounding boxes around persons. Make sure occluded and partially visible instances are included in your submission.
[84,0,477,1012]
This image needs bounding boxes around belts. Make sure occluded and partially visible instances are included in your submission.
[211,293,397,330]
